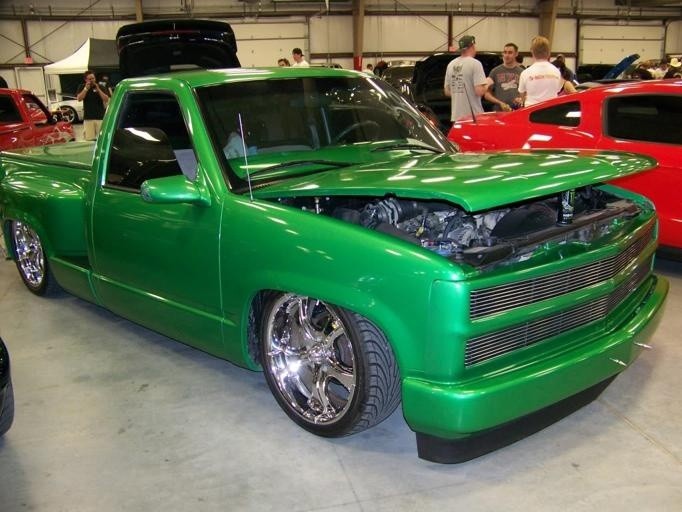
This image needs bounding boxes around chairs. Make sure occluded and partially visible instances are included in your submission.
[252,104,314,145]
[111,126,183,189]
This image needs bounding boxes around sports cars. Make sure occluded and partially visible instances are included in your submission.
[445,79,682,254]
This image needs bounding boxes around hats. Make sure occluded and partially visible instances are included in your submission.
[457,36,475,52]
[668,58,681,67]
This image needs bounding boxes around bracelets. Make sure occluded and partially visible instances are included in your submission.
[84,87,89,92]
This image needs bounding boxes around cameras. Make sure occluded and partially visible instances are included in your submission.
[89,80,96,89]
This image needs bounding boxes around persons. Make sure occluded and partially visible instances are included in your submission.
[362,63,373,74]
[484,42,525,111]
[552,61,578,96]
[620,57,682,80]
[292,47,310,68]
[77,70,110,140]
[99,74,112,96]
[518,35,563,107]
[277,58,291,67]
[556,54,573,82]
[444,35,494,123]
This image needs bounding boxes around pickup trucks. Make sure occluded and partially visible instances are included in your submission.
[2,65,672,445]
[0,85,78,159]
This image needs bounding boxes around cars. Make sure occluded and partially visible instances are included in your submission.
[48,98,84,125]
